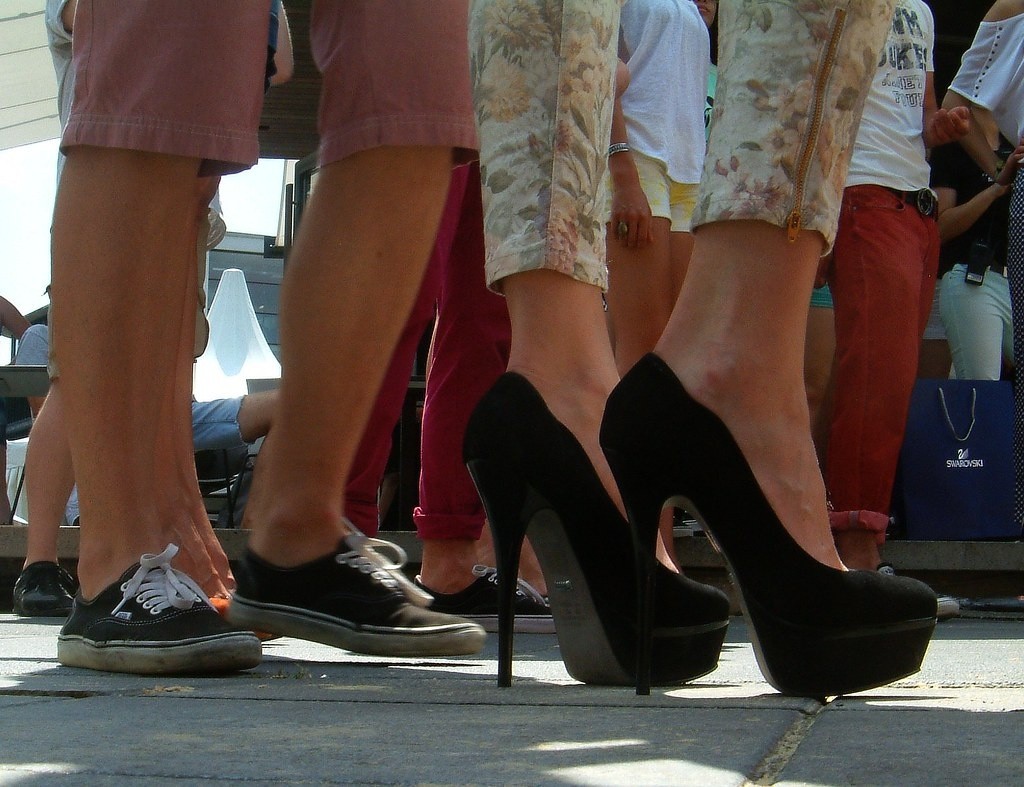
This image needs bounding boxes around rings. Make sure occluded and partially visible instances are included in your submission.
[618,222,627,237]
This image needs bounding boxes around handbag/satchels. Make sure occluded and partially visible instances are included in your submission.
[892,377,1022,541]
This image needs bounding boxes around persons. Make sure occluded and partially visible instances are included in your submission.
[49,0,484,679]
[8,187,284,617]
[605,0,710,571]
[918,102,1015,381]
[460,0,943,695]
[695,0,721,154]
[943,2,1024,544]
[341,149,553,616]
[803,0,969,619]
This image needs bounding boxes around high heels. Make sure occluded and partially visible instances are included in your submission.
[468,371,733,688]
[598,354,937,696]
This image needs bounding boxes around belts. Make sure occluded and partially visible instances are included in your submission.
[882,184,940,223]
[957,251,1011,279]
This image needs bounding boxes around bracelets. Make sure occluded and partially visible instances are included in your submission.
[993,159,1006,184]
[608,142,631,159]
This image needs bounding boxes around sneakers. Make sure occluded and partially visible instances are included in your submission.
[406,567,556,633]
[228,533,485,653]
[877,566,959,622]
[59,545,263,673]
[12,561,76,619]
